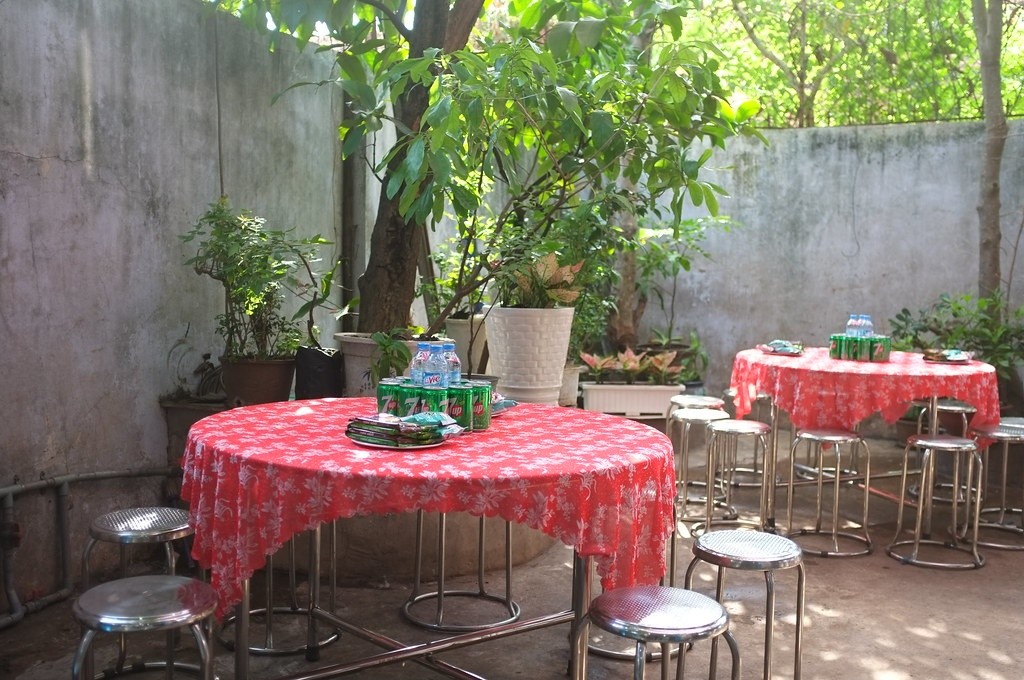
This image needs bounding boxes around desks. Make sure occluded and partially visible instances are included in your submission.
[727,346,1000,541]
[179,396,679,680]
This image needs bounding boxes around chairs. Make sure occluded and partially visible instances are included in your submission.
[664,391,1024,571]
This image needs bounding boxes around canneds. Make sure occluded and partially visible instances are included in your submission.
[376,375,493,434]
[828,333,892,363]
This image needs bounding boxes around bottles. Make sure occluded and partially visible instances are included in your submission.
[422,345,449,388]
[443,343,462,385]
[857,315,865,337]
[861,315,873,337]
[409,343,430,386]
[846,315,858,337]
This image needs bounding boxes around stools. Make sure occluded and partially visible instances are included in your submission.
[675,529,807,680]
[69,575,220,680]
[212,518,341,656]
[81,507,195,652]
[570,586,742,680]
[401,507,520,635]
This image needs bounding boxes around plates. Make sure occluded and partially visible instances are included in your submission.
[763,351,803,357]
[491,410,510,418]
[351,438,445,449]
[925,360,973,365]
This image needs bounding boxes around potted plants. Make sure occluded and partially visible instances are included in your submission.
[159,321,227,465]
[332,327,456,397]
[444,306,487,373]
[289,268,344,401]
[648,326,710,396]
[578,344,685,420]
[482,250,585,403]
[888,291,1024,451]
[175,193,336,405]
[558,262,622,408]
